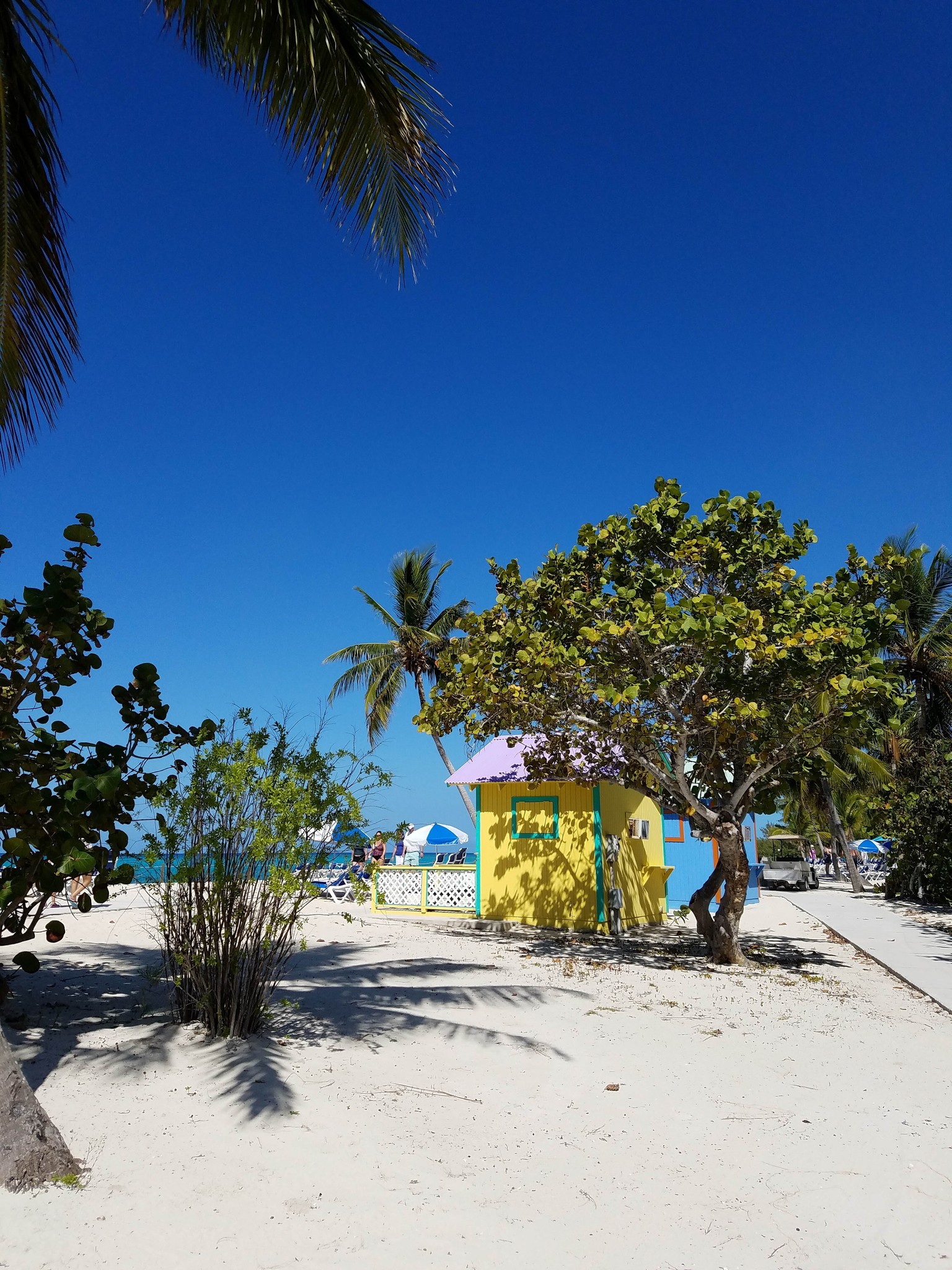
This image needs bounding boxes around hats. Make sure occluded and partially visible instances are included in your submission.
[408,823,414,829]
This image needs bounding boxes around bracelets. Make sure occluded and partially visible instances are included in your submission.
[421,851,423,853]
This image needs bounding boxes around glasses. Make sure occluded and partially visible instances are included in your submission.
[400,833,402,835]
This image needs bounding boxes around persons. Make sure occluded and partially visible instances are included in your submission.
[824,843,831,877]
[842,850,859,870]
[861,852,868,864]
[351,831,385,875]
[44,871,92,909]
[391,823,423,874]
[808,844,817,859]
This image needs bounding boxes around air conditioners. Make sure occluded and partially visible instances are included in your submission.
[742,825,751,842]
[629,818,649,839]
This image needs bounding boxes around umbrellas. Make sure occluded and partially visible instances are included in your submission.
[297,818,372,898]
[848,839,890,863]
[403,822,469,857]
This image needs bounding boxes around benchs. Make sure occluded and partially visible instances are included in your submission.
[776,857,804,862]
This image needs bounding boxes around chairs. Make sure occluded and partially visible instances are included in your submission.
[31,850,118,897]
[806,857,889,881]
[281,858,395,904]
[432,847,468,866]
